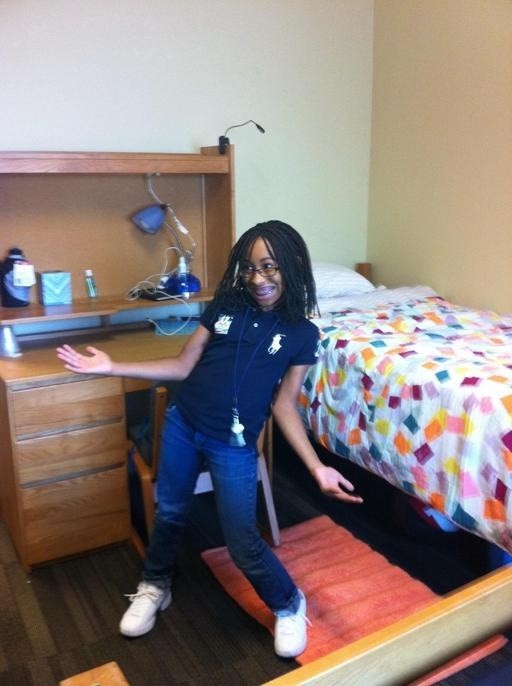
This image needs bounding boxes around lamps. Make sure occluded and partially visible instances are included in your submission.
[127,203,201,337]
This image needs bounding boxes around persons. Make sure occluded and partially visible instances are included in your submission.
[57,219,362,657]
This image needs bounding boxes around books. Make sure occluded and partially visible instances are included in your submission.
[131,287,182,301]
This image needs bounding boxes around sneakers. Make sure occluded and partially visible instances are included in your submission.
[273,588,308,659]
[119,582,173,637]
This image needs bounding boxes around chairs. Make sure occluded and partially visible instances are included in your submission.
[126,365,282,575]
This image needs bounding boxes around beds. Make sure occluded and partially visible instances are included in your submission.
[244,264,512,567]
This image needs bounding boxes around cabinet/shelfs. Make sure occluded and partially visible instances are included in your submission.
[1,144,237,576]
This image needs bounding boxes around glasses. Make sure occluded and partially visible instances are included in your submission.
[237,266,280,278]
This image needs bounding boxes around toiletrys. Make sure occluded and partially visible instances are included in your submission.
[84,270,96,298]
[0,247,32,307]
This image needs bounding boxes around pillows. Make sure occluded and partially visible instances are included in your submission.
[289,268,375,298]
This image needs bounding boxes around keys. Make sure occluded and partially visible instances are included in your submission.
[230,411,247,448]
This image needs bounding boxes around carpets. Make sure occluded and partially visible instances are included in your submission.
[199,514,510,684]
